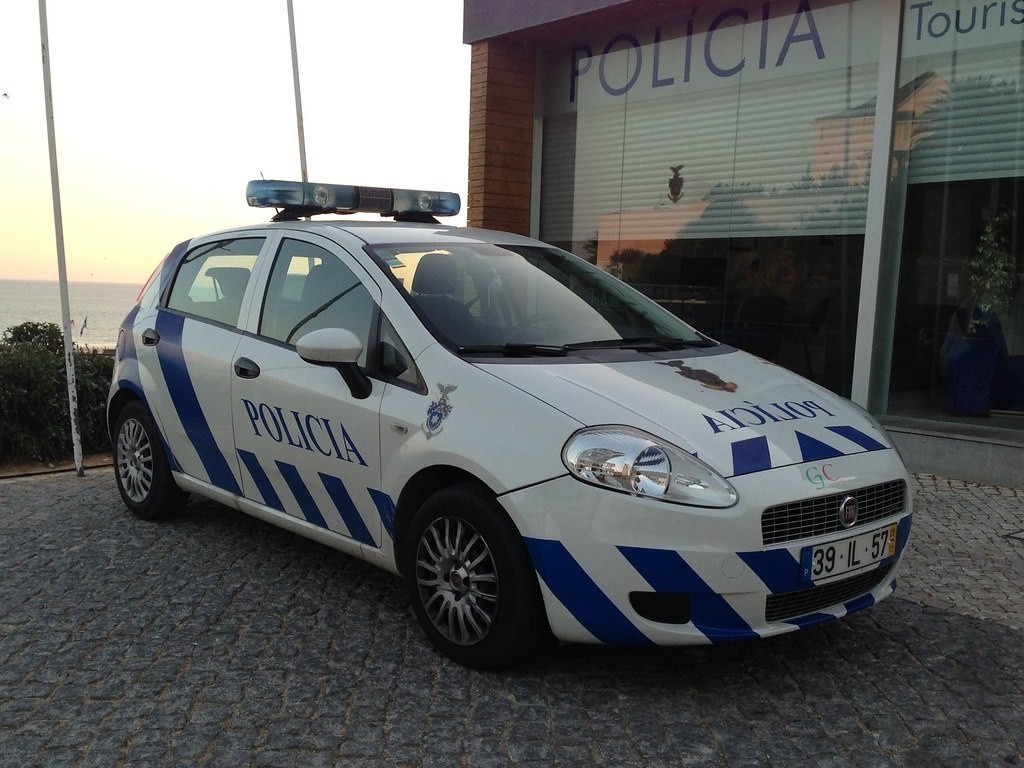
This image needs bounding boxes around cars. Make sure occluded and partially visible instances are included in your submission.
[106,180,913,672]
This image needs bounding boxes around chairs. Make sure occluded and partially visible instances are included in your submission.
[205,267,252,326]
[411,254,480,347]
[286,264,372,345]
